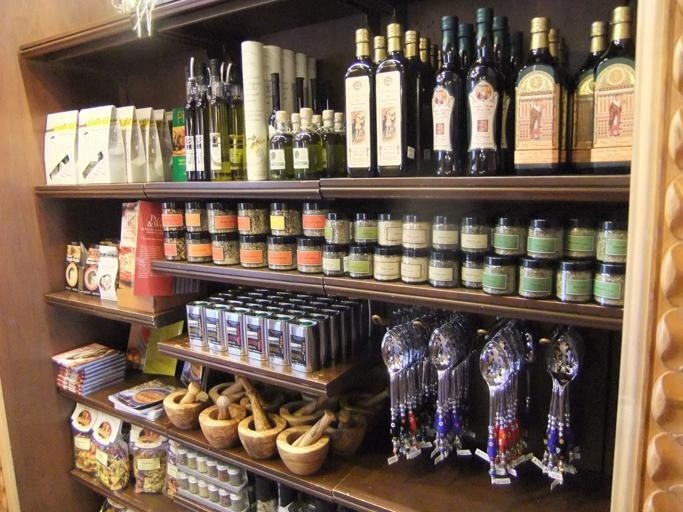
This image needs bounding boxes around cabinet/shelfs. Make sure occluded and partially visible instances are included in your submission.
[0,1,683,511]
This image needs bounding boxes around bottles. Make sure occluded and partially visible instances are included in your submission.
[431,5,524,178]
[344,23,441,179]
[263,107,347,182]
[513,15,572,176]
[591,5,636,173]
[184,55,245,181]
[575,20,607,174]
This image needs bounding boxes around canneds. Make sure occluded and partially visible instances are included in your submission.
[185,287,366,373]
